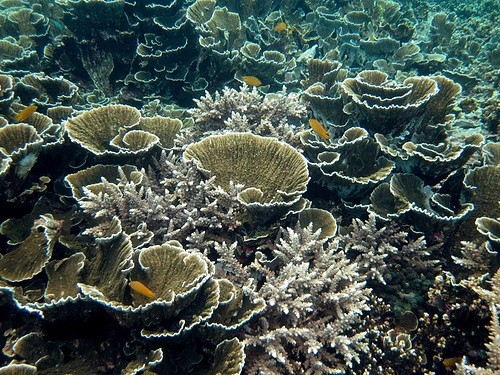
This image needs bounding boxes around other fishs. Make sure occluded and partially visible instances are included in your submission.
[243,76,262,86]
[442,356,462,368]
[274,22,286,31]
[14,106,38,122]
[308,118,330,139]
[128,281,156,298]
